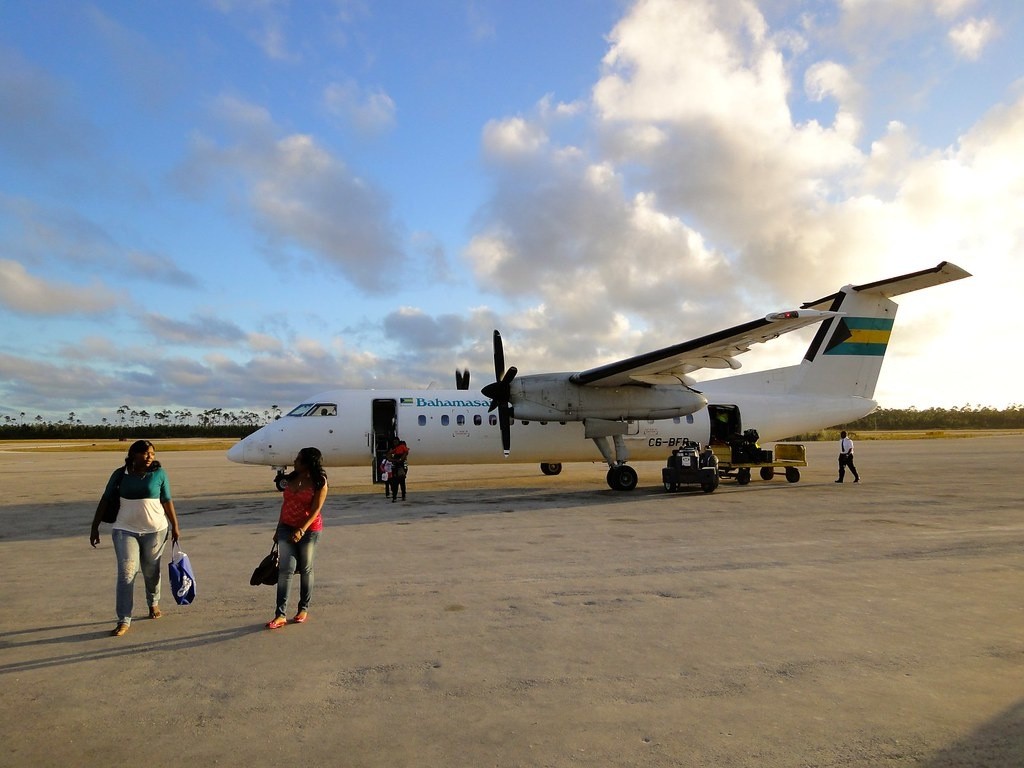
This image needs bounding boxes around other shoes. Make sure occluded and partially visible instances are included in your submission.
[267,616,286,628]
[294,610,308,623]
[111,624,128,636]
[149,609,161,618]
[392,500,396,502]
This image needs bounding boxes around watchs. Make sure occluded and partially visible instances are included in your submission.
[299,527,305,537]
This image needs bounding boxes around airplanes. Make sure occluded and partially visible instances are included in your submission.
[224,261,972,493]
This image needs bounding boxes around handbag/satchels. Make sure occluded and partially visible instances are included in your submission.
[250,541,279,586]
[168,541,196,605]
[101,466,126,523]
[381,473,388,481]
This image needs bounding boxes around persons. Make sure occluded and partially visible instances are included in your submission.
[90,439,178,636]
[670,441,711,467]
[267,446,329,628]
[320,409,329,416]
[381,437,410,503]
[835,431,860,483]
